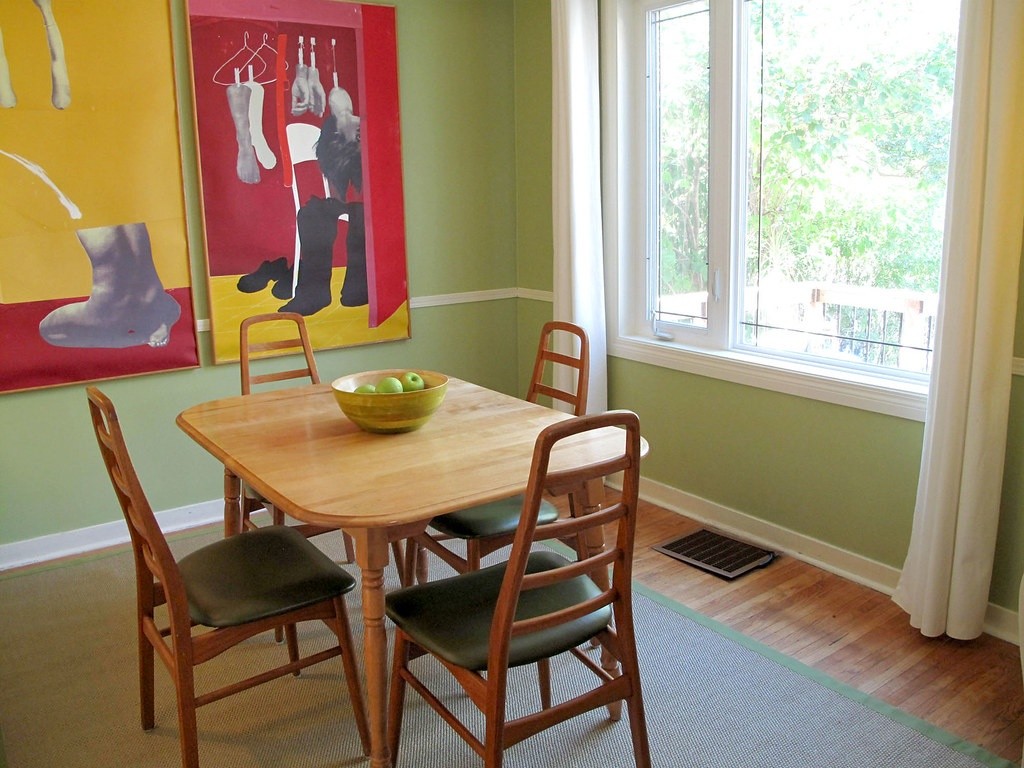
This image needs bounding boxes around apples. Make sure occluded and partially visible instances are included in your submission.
[354,372,425,393]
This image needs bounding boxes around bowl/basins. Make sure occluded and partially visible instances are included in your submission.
[330,369,449,435]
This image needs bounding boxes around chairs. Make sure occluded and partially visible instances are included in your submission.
[240,312,405,645]
[377,408,658,768]
[409,319,591,583]
[85,382,366,768]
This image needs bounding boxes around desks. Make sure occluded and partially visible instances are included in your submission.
[177,376,648,768]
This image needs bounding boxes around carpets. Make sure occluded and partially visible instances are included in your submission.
[0,519,1018,768]
